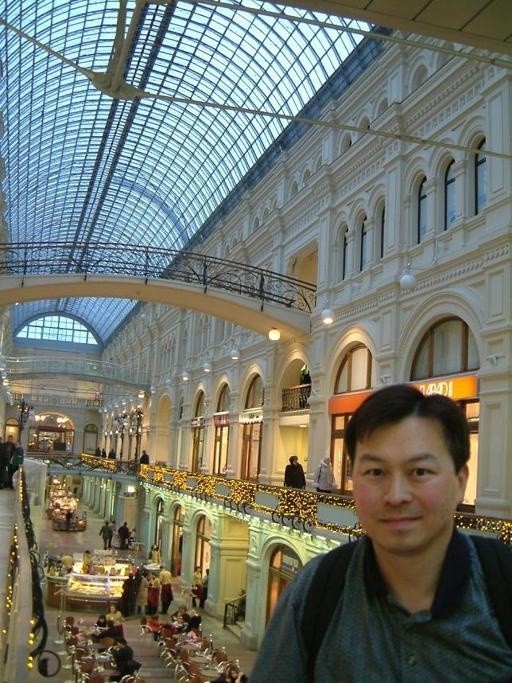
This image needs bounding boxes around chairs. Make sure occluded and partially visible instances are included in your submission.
[140,611,248,683]
[56,615,140,681]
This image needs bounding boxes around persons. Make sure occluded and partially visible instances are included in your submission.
[101,448,105,457]
[140,449,149,464]
[248,384,511,683]
[234,588,247,625]
[108,447,116,458]
[284,454,307,490]
[228,665,248,683]
[313,457,337,493]
[205,664,245,683]
[0,435,26,489]
[63,507,210,683]
[95,446,101,455]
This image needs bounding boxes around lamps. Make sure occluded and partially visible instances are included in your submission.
[399,271,417,291]
[322,308,336,325]
[230,348,240,360]
[267,326,282,341]
[203,361,212,373]
